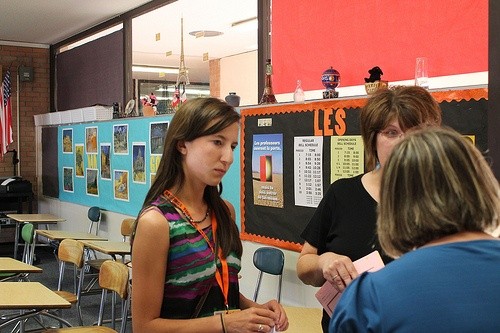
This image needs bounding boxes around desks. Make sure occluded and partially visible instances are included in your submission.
[0,257,43,283]
[75,241,131,327]
[6,213,66,264]
[0,281,71,333]
[273,305,326,333]
[29,229,108,291]
[43,327,119,333]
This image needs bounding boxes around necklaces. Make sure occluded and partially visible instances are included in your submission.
[159,192,210,224]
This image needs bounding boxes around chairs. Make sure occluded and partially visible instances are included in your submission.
[0,207,137,333]
[252,247,285,305]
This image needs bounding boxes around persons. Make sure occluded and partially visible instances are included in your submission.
[328,124,500,333]
[129,97,290,333]
[295,86,443,333]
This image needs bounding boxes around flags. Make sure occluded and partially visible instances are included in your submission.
[0,66,14,164]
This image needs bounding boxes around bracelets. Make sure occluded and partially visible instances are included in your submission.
[220,313,226,333]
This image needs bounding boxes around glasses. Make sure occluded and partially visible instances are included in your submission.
[378,129,405,143]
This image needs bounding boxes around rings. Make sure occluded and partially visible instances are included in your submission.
[257,324,263,332]
[330,275,339,280]
